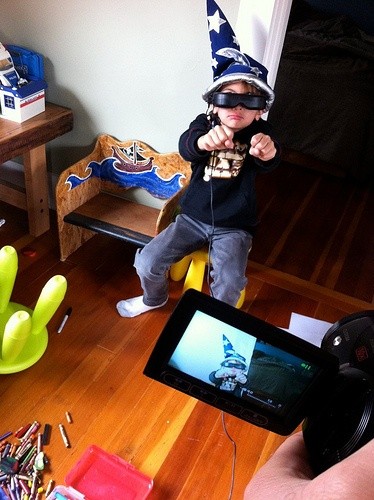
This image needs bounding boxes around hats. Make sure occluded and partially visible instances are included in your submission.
[202,0,275,113]
[221,334,247,372]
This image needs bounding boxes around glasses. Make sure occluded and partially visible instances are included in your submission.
[224,363,243,369]
[213,92,266,110]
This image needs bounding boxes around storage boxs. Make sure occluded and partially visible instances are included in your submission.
[0,78,49,122]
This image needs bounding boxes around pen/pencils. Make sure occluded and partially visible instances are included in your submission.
[57,307,73,334]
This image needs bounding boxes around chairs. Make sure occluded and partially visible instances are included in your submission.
[55,135,194,261]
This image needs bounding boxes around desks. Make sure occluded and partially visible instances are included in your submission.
[0,101,74,238]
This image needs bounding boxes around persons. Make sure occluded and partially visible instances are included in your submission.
[243,430,374,500]
[209,334,248,395]
[116,44,280,318]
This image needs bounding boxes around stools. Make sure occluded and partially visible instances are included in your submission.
[170,240,252,309]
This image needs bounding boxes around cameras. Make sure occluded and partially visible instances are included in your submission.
[142,286,373,479]
